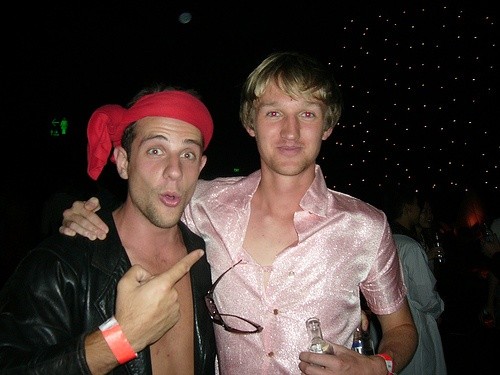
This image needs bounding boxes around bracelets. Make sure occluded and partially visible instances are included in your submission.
[376,352,395,374]
[98,315,138,364]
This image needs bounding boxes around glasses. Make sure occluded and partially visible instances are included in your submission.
[202,259,263,334]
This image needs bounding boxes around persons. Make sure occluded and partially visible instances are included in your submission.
[0,89,217,375]
[381,185,500,375]
[60,52,418,375]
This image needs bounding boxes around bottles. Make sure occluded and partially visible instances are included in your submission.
[305,317,335,369]
[351,324,375,356]
[482,221,494,241]
[433,232,443,263]
